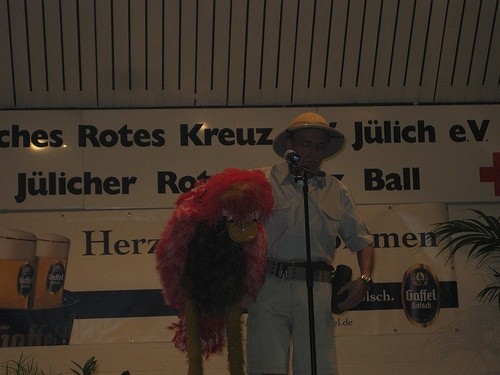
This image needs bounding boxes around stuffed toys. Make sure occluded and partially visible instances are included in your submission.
[155,168,277,374]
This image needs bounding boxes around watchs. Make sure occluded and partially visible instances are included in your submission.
[357,273,374,290]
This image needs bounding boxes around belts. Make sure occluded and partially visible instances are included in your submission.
[263,260,331,282]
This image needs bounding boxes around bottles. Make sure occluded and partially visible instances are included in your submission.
[330,265,352,314]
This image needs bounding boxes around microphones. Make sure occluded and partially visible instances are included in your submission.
[284,150,301,163]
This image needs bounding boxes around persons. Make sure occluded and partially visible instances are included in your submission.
[248,110,375,374]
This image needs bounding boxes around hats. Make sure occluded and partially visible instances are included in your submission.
[272,111,345,159]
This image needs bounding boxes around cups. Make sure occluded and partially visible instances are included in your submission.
[0,236,37,309]
[34,238,72,307]
